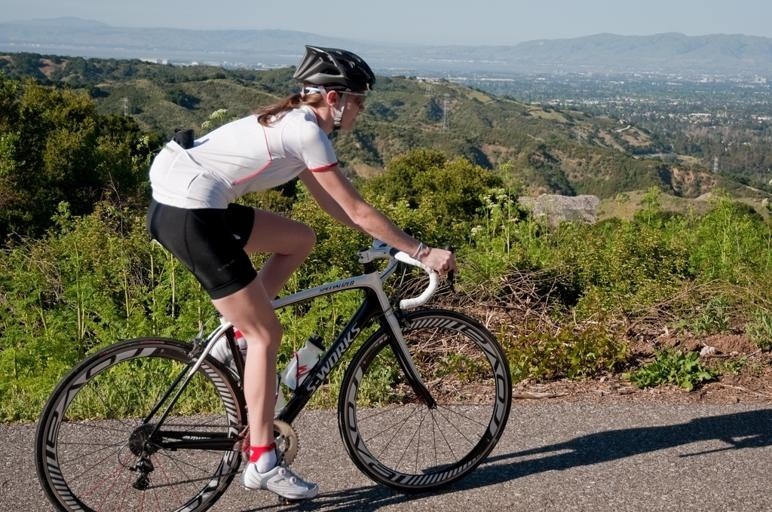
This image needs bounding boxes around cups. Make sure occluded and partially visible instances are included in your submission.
[281,336,327,390]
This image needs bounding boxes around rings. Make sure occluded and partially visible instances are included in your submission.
[445,265,451,272]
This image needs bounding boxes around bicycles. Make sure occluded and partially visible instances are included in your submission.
[33,232,512,512]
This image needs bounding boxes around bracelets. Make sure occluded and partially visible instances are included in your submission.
[411,240,424,260]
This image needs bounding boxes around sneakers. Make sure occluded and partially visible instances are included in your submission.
[240,461,319,501]
[210,337,246,382]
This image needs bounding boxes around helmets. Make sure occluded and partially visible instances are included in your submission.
[293,45,376,98]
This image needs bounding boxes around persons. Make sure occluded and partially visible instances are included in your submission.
[143,44,460,502]
